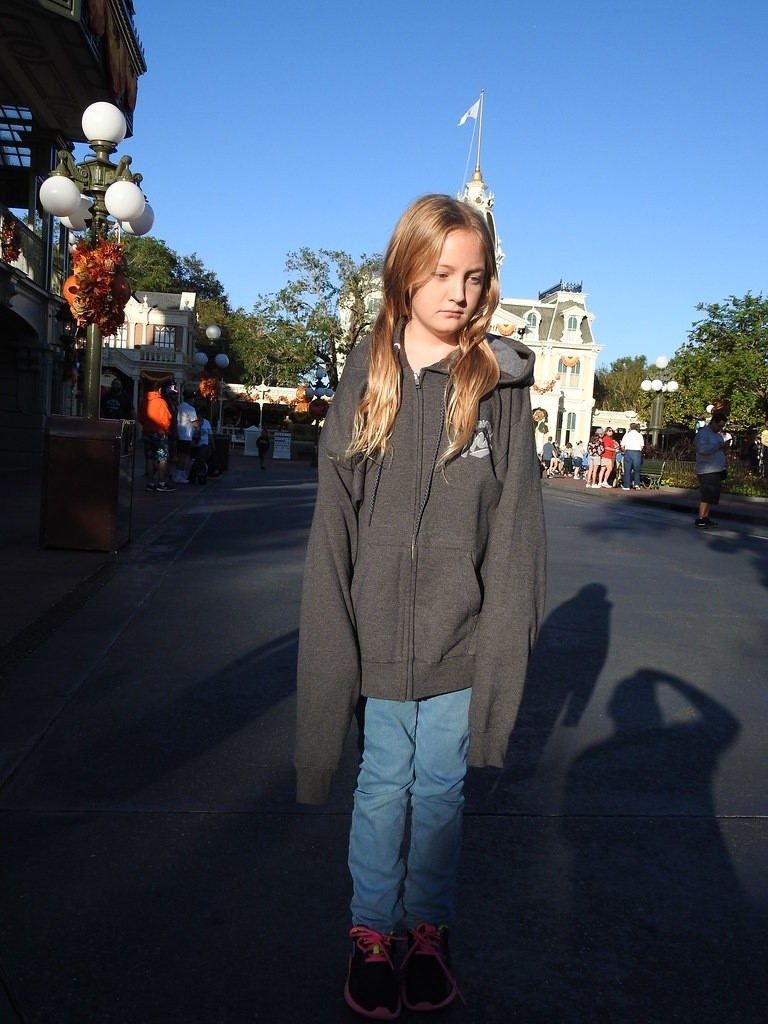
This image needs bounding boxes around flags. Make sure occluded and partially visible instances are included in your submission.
[457,99,480,126]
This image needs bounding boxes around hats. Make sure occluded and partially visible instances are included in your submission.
[595,428,603,433]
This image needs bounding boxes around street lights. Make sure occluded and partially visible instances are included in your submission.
[640,356,679,459]
[192,325,230,432]
[304,368,335,468]
[38,101,155,418]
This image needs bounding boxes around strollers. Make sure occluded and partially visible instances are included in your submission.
[607,452,635,489]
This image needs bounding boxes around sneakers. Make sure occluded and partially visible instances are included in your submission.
[344,924,401,1016]
[400,923,461,1009]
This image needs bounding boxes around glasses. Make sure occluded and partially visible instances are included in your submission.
[717,423,724,427]
[609,430,613,432]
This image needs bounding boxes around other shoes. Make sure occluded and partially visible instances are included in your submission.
[636,487,640,490]
[695,516,719,528]
[622,488,630,491]
[586,484,591,488]
[156,482,176,491]
[591,484,598,488]
[602,482,612,488]
[598,483,602,488]
[174,475,190,483]
[145,483,155,491]
[573,476,580,479]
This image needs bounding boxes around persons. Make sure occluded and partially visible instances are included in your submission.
[239,427,243,435]
[292,195,548,1017]
[256,429,269,470]
[101,378,136,419]
[620,423,645,491]
[692,412,729,529]
[138,378,218,492]
[538,426,624,488]
